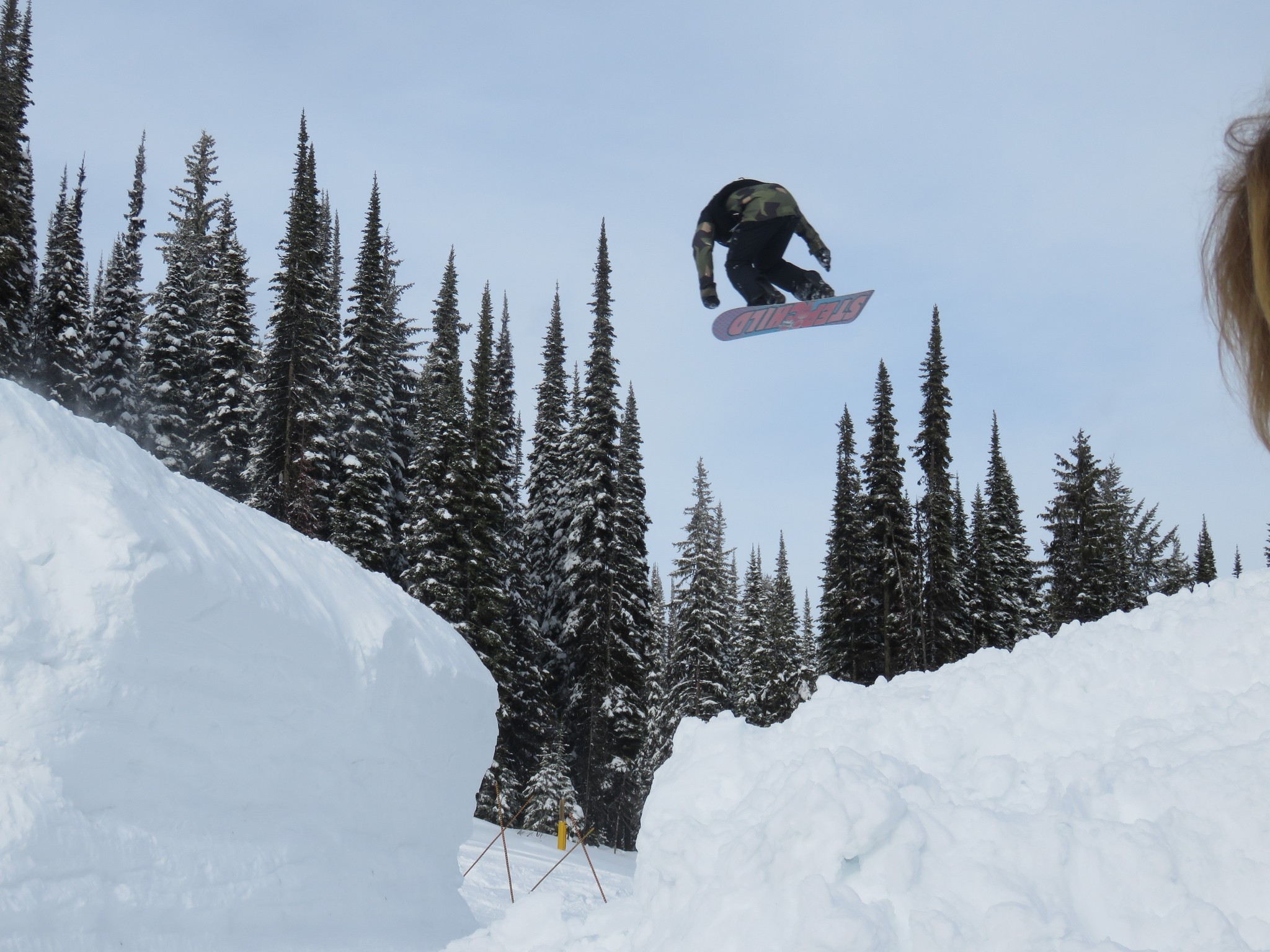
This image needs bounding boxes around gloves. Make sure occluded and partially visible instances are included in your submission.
[700,282,720,309]
[814,247,831,272]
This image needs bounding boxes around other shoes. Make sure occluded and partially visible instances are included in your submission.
[747,291,785,308]
[810,284,834,300]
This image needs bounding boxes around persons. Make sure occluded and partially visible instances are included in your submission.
[691,177,835,309]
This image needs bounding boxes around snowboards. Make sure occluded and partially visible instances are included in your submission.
[712,289,875,341]
